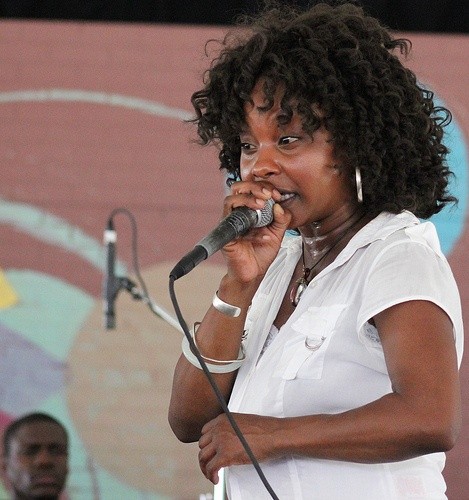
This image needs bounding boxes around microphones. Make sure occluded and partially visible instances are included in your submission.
[103,218,118,330]
[170,193,276,281]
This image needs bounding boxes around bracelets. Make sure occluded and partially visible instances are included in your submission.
[213,290,253,318]
[179,320,247,375]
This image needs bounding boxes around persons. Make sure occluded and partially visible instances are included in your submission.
[168,4,464,500]
[3,413,70,500]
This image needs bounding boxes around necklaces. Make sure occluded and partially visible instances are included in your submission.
[289,237,342,307]
